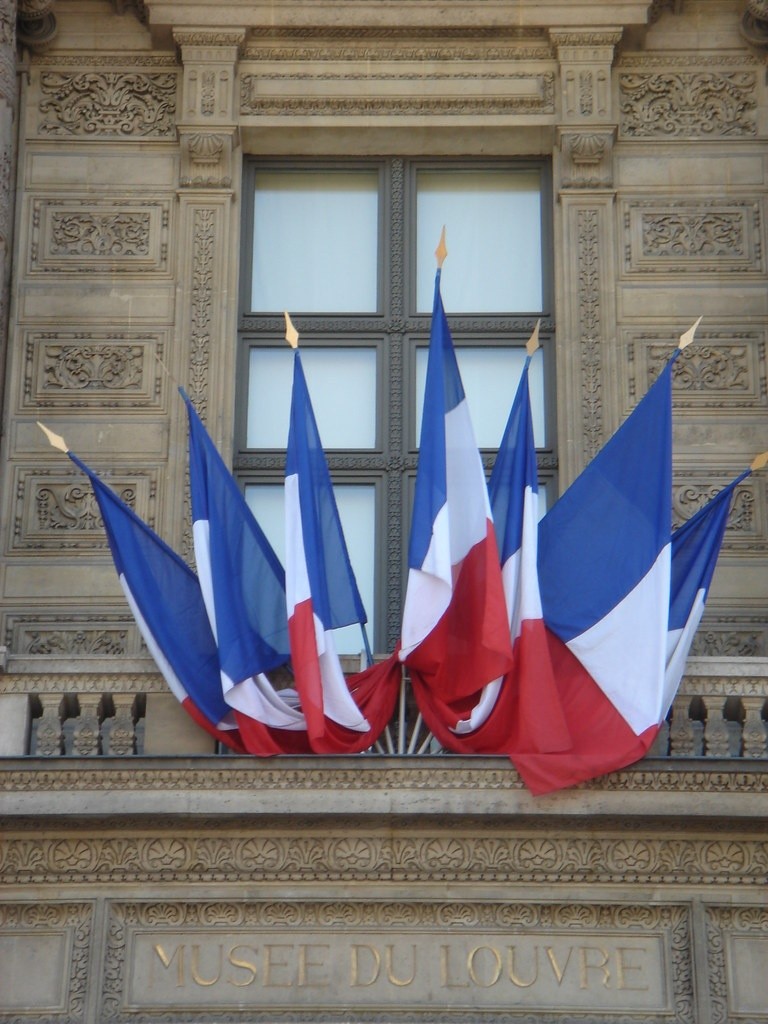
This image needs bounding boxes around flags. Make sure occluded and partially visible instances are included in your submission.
[393,269,513,704]
[511,349,684,795]
[178,386,309,756]
[659,464,751,734]
[285,350,403,754]
[411,356,574,752]
[66,449,245,754]
